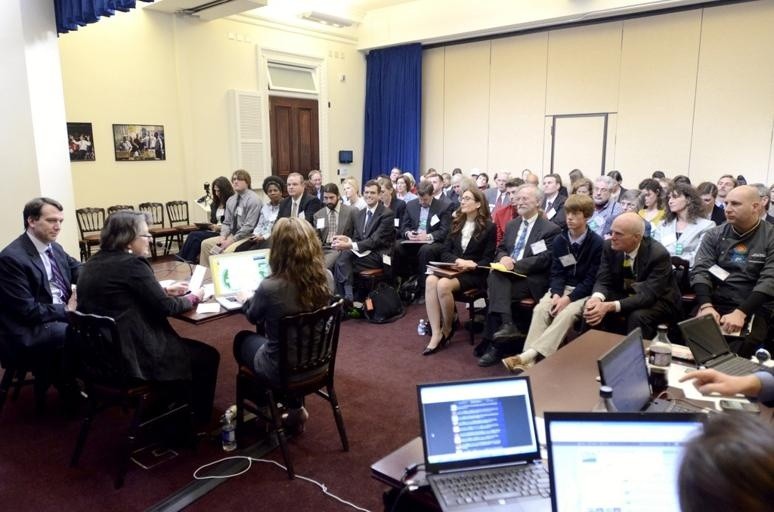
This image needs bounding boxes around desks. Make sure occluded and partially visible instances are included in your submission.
[370,328,774,512]
[149,260,245,326]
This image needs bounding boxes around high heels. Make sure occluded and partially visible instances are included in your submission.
[281,406,309,433]
[422,332,445,355]
[443,327,455,344]
[255,408,273,434]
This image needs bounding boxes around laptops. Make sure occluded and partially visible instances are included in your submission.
[543,411,708,512]
[598,326,704,413]
[417,375,552,512]
[677,313,768,377]
[208,248,273,311]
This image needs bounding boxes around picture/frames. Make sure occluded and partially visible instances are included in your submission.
[67,121,95,162]
[113,124,166,161]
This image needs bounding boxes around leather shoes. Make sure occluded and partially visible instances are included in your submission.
[478,347,501,367]
[492,322,521,342]
[334,294,353,312]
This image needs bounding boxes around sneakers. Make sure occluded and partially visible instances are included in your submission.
[502,355,522,372]
[513,363,529,373]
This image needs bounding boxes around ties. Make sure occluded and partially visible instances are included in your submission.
[365,211,372,234]
[232,195,240,235]
[623,255,637,297]
[46,247,69,302]
[572,242,578,257]
[291,203,297,217]
[326,211,337,244]
[495,193,502,215]
[546,202,551,212]
[512,221,528,261]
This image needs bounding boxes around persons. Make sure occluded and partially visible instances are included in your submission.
[184,172,773,310]
[422,189,497,357]
[678,410,773,511]
[116,126,164,159]
[68,129,94,161]
[234,217,335,432]
[500,195,602,372]
[0,198,86,406]
[689,185,773,359]
[583,212,680,335]
[477,184,561,365]
[679,367,773,410]
[77,208,220,437]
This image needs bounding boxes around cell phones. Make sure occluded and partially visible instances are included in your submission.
[720,400,762,414]
[412,231,419,235]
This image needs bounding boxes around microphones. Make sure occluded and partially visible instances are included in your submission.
[156,241,192,277]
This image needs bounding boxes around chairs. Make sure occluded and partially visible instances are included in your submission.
[108,205,154,261]
[66,311,196,489]
[76,207,105,263]
[680,293,698,302]
[139,203,182,260]
[0,360,54,417]
[512,296,536,326]
[453,287,487,345]
[167,201,200,255]
[235,298,349,479]
[355,269,383,293]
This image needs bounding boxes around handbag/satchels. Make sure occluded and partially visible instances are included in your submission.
[401,275,422,306]
[368,286,403,321]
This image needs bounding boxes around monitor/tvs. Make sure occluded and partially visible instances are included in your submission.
[339,151,353,164]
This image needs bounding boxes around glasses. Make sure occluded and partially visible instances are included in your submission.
[461,195,478,203]
[138,233,152,239]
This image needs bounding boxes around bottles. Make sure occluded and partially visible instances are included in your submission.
[417,319,426,336]
[649,324,672,395]
[593,385,618,412]
[220,410,237,452]
